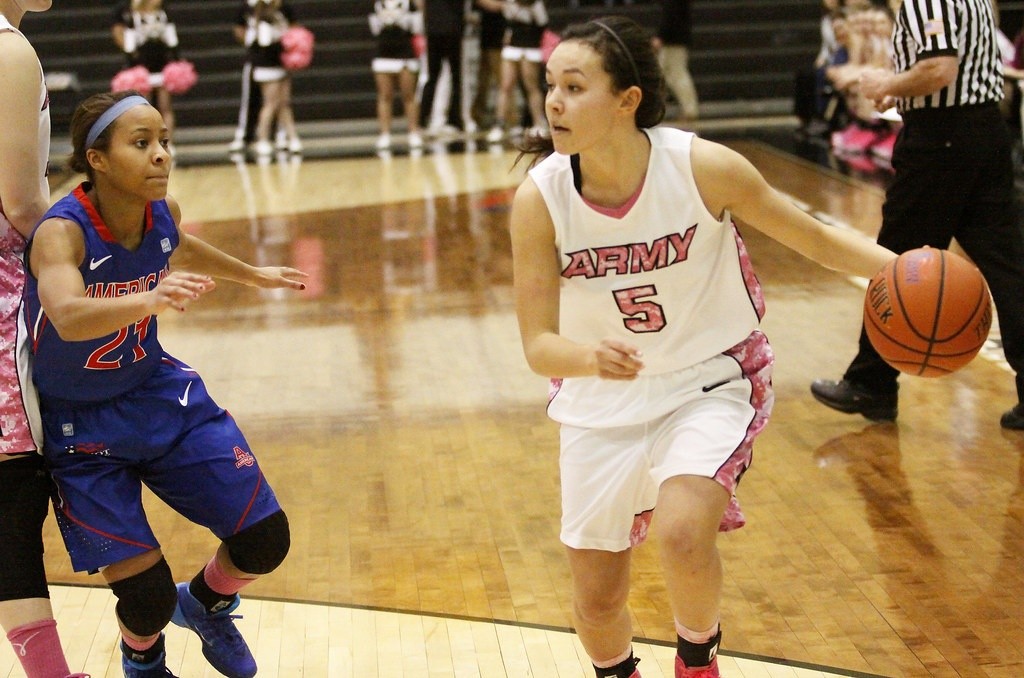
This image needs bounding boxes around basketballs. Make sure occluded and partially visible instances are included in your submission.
[863,246,993,377]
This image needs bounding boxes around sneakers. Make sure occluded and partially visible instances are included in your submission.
[119,632,178,678]
[811,378,898,422]
[170,581,257,678]
[1000,403,1024,430]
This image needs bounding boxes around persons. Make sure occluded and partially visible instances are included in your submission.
[510,15,931,678]
[0,0,311,678]
[792,0,1024,432]
[366,1,698,150]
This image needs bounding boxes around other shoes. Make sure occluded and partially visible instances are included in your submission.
[675,655,721,678]
[65,672,90,678]
[275,130,287,148]
[592,659,641,678]
[409,132,421,147]
[510,126,522,133]
[252,139,273,154]
[227,139,245,150]
[376,132,390,148]
[487,127,503,141]
[527,122,548,138]
[288,138,302,151]
